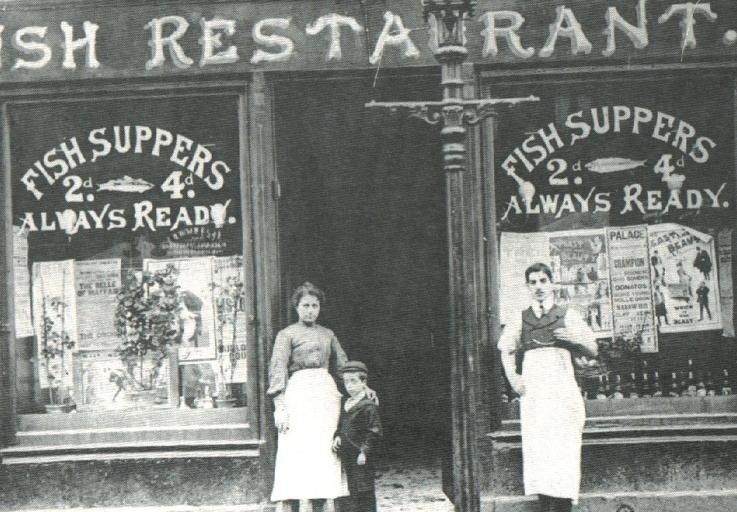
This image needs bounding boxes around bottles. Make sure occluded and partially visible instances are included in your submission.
[582,369,736,400]
[500,377,519,404]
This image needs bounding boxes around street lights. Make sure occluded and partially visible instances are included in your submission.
[363,0,541,512]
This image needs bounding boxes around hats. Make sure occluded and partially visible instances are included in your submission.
[341,360,369,373]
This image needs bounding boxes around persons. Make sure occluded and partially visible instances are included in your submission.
[652,246,712,326]
[331,360,383,512]
[266,281,380,512]
[497,262,599,512]
